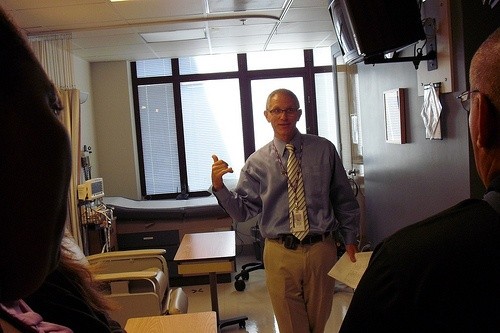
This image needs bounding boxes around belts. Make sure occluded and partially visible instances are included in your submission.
[300,231,331,245]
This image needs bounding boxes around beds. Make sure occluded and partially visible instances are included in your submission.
[104,193,232,242]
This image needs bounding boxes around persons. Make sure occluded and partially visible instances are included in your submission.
[0,7,128,333]
[339,29,500,333]
[211,89,361,333]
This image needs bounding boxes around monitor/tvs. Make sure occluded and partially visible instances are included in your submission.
[327,0,425,66]
[78,178,103,202]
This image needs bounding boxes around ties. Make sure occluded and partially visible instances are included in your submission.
[283,143,310,242]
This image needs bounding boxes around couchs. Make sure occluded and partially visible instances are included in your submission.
[87,249,188,329]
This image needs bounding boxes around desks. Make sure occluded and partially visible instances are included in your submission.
[174,231,249,330]
[124,311,217,333]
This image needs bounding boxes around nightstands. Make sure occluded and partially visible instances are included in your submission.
[83,216,119,254]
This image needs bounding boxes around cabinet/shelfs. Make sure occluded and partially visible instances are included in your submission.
[118,229,182,285]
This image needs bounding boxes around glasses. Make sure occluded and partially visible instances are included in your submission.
[455,88,496,115]
[267,107,298,116]
